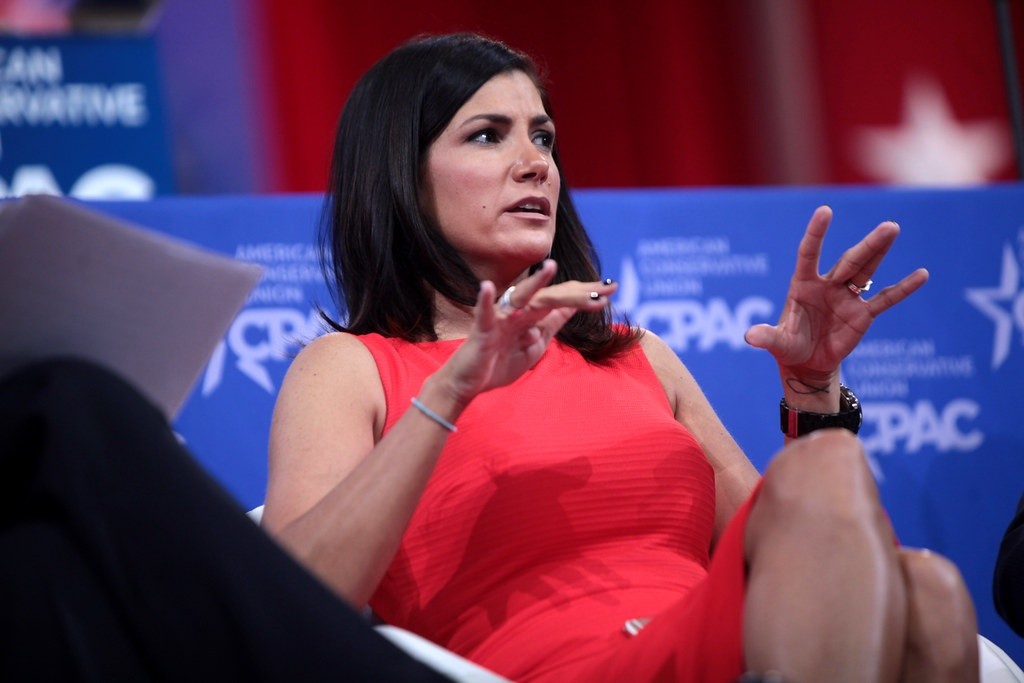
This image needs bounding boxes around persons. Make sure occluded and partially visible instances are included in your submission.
[261,35,982,682]
[1,350,463,683]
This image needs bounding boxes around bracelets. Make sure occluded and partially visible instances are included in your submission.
[410,396,458,433]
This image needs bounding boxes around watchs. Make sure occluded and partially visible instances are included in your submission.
[780,383,863,437]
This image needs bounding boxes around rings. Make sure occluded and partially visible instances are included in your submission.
[846,280,863,296]
[497,285,519,315]
